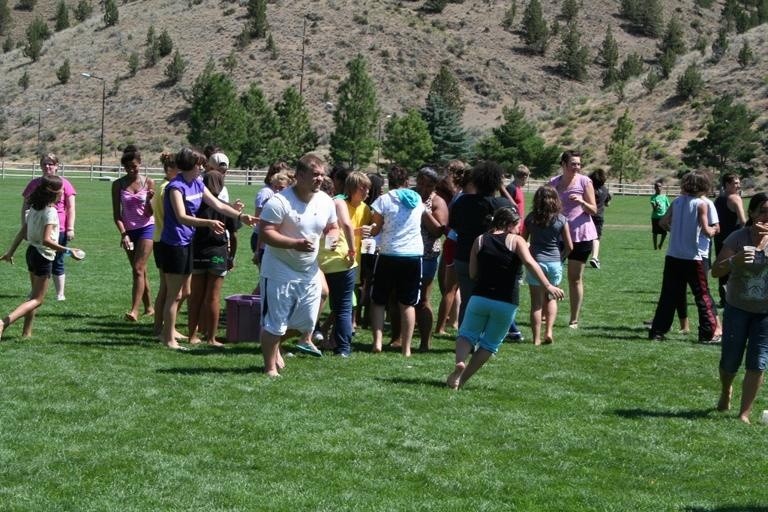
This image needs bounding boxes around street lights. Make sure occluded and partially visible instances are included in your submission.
[36,108,52,166]
[82,73,107,180]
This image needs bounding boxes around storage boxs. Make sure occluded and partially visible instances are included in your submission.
[225,293,263,343]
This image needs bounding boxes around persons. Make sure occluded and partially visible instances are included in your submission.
[156,150,263,350]
[112,145,155,321]
[22,153,77,300]
[650,172,768,424]
[0,175,84,342]
[250,151,612,393]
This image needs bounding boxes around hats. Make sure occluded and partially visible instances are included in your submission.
[209,152,229,170]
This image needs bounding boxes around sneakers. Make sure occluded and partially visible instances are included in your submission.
[503,331,524,343]
[589,256,600,269]
[314,331,325,342]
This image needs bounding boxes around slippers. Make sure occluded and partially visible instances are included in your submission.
[295,343,322,357]
